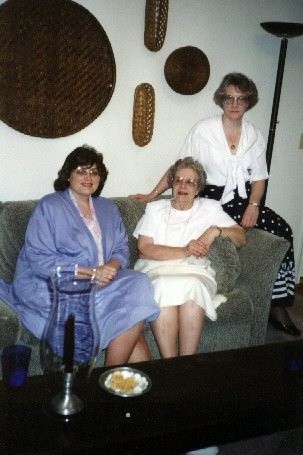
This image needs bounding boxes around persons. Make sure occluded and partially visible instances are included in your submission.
[0,147,161,366]
[129,72,301,336]
[132,156,245,359]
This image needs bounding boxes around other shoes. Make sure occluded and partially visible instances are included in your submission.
[269,308,301,336]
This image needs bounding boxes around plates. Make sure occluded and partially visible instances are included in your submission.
[99,367,152,397]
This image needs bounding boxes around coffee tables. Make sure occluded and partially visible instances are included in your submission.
[0,340,303,455]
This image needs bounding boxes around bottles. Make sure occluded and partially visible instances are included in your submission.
[39,263,99,392]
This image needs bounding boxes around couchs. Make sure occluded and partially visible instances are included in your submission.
[0,195,291,377]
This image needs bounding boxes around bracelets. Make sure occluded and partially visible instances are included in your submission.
[154,190,159,194]
[249,203,259,207]
[217,227,222,237]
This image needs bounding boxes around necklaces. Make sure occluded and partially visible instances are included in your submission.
[228,133,237,149]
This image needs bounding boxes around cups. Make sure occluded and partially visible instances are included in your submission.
[3,345,31,388]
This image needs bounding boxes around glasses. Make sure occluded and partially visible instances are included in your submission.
[73,168,102,179]
[223,95,248,106]
[173,176,200,188]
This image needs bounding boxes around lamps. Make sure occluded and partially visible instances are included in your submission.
[259,21,303,210]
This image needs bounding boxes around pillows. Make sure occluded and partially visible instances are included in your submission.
[207,236,241,294]
